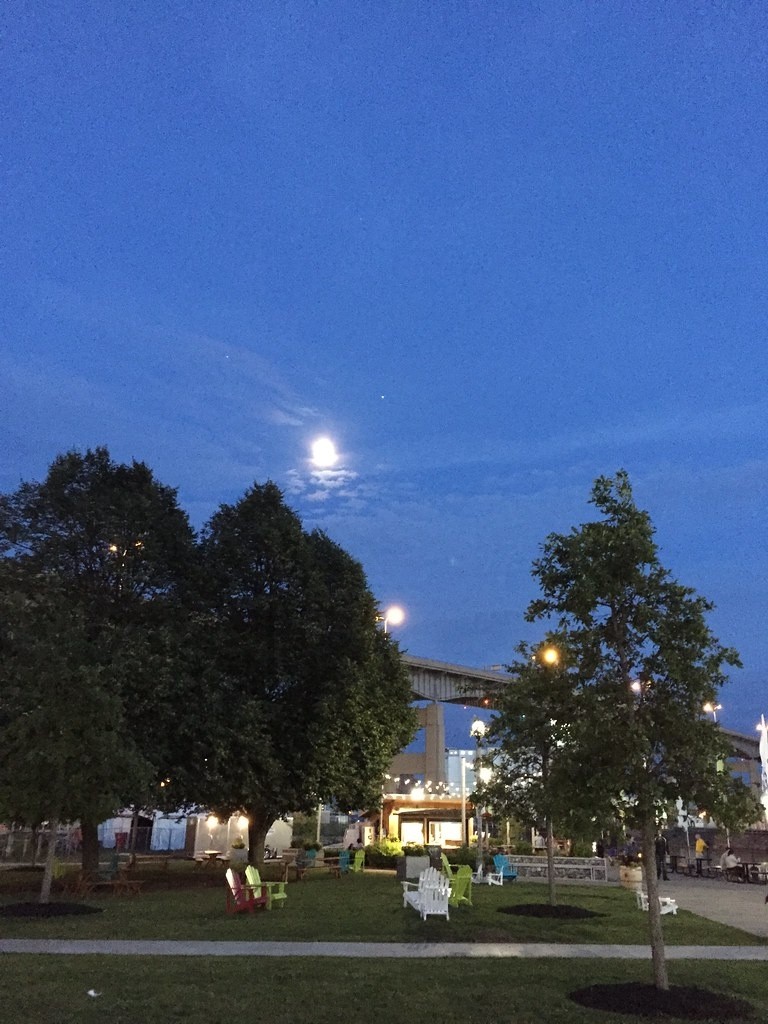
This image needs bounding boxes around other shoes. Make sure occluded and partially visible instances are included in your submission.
[663,878,670,881]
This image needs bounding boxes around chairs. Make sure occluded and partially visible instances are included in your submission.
[440,852,470,884]
[337,849,350,871]
[486,865,505,887]
[245,865,288,911]
[449,865,473,908]
[636,891,679,915]
[349,849,365,872]
[398,867,450,921]
[225,868,267,915]
[472,864,483,884]
[493,853,518,883]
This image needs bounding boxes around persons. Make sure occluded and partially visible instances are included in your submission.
[265,845,272,859]
[719,846,745,883]
[481,845,509,872]
[693,834,707,880]
[347,843,355,851]
[655,829,671,881]
[532,831,546,855]
[223,838,249,864]
[595,839,640,866]
[355,838,363,850]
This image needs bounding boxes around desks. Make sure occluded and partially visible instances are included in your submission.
[317,856,350,873]
[136,855,176,872]
[737,862,761,884]
[204,850,224,871]
[687,858,713,879]
[265,859,292,882]
[74,869,138,897]
[667,855,685,875]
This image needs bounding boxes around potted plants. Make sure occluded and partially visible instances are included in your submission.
[303,841,322,860]
[616,852,646,889]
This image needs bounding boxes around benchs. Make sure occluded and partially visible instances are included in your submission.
[136,862,172,874]
[221,858,232,870]
[668,863,740,884]
[62,880,144,900]
[192,857,204,873]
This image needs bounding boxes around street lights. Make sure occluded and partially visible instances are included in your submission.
[471,720,487,870]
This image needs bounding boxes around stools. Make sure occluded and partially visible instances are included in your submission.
[297,868,313,879]
[329,866,343,880]
